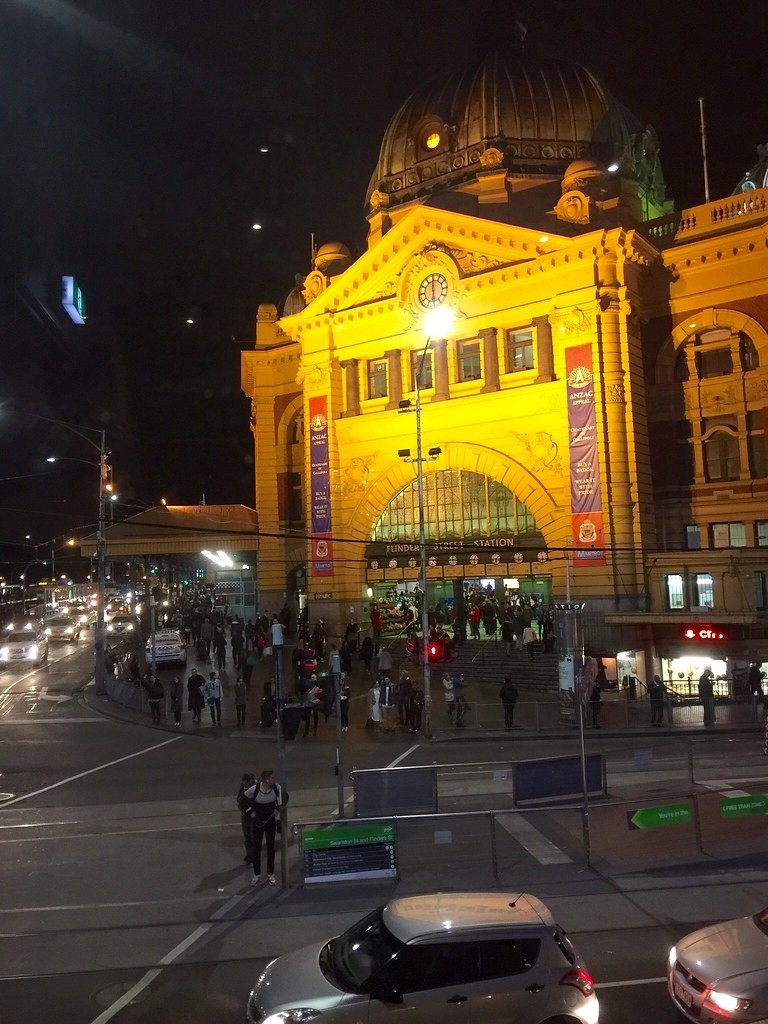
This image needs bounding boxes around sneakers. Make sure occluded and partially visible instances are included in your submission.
[267,873,275,886]
[250,874,260,887]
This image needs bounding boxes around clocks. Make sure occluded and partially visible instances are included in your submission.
[536,551,547,563]
[408,558,417,568]
[469,554,479,565]
[427,556,437,567]
[491,554,501,565]
[388,559,398,569]
[371,561,378,569]
[418,273,449,309]
[513,552,523,563]
[449,556,458,566]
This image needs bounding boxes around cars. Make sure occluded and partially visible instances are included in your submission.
[0,581,180,669]
[244,890,600,1024]
[144,627,188,669]
[667,904,768,1024]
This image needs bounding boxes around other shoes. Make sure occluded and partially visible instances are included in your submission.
[174,722,181,729]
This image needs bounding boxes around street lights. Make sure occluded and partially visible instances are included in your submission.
[412,309,457,735]
[47,457,110,696]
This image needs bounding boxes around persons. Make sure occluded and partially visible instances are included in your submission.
[750,662,766,695]
[500,678,518,730]
[234,677,246,727]
[404,593,544,660]
[256,604,425,737]
[699,670,715,725]
[187,669,206,721]
[647,675,664,728]
[237,774,257,869]
[171,676,183,727]
[158,584,256,671]
[592,680,602,728]
[205,672,223,726]
[132,658,140,685]
[148,675,160,725]
[442,672,465,727]
[244,770,282,887]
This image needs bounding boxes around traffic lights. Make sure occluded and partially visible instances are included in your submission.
[429,645,438,656]
